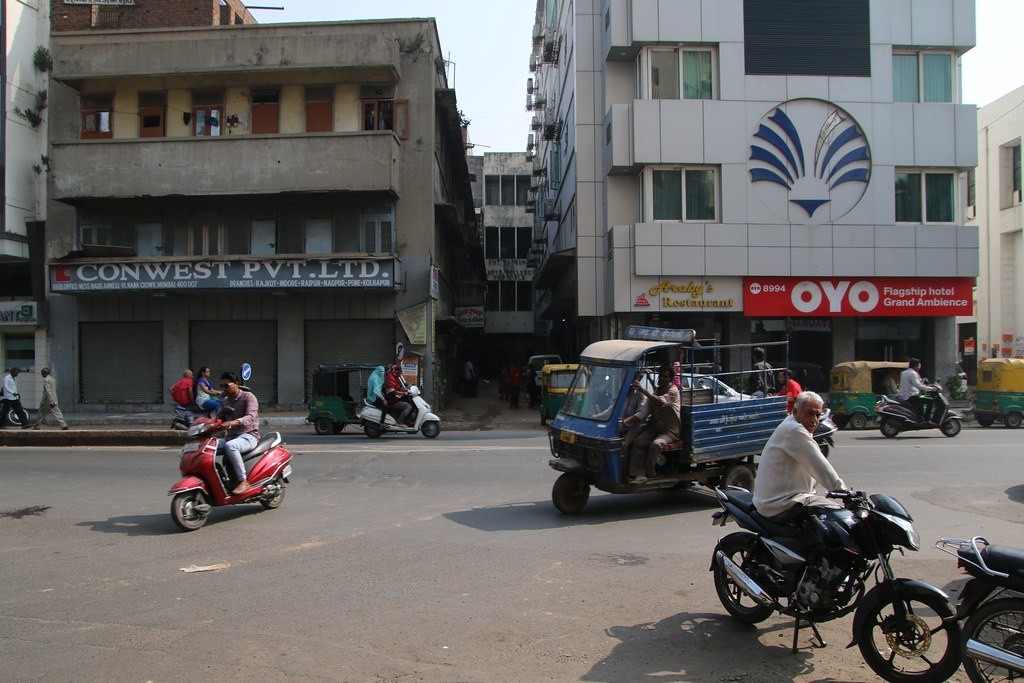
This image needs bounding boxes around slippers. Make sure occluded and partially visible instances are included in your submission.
[629,477,647,485]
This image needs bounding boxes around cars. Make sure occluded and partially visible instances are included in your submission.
[634,373,759,403]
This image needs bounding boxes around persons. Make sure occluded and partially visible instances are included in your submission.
[30,367,69,430]
[499,365,508,399]
[508,360,522,410]
[586,376,650,474]
[0,367,33,429]
[190,370,260,495]
[674,362,685,390]
[378,110,391,129]
[753,391,855,524]
[899,358,937,425]
[525,364,538,407]
[194,367,222,418]
[883,369,899,398]
[777,368,803,414]
[170,369,195,406]
[384,364,412,427]
[367,366,389,426]
[461,355,481,398]
[748,347,775,397]
[628,364,682,484]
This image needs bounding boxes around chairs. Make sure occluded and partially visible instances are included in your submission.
[874,375,895,399]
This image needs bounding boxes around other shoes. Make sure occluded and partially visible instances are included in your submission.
[22,424,32,429]
[380,422,390,428]
[818,587,847,615]
[920,420,934,426]
[63,427,70,430]
[396,422,407,428]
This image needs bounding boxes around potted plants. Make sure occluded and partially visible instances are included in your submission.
[945,374,963,400]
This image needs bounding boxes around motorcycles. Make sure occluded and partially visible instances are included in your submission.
[709,482,968,683]
[539,363,591,426]
[972,357,1024,430]
[547,324,791,515]
[933,536,1024,683]
[831,361,920,431]
[304,361,389,436]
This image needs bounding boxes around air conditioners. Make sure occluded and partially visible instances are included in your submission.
[545,199,553,215]
[534,224,543,240]
[528,134,533,149]
[537,0,544,12]
[531,238,538,251]
[545,30,554,51]
[530,54,536,71]
[533,157,541,174]
[527,147,533,157]
[526,189,534,202]
[527,80,533,94]
[543,45,552,62]
[545,107,554,128]
[527,95,532,110]
[529,248,537,260]
[530,175,538,189]
[532,116,539,130]
[532,24,541,45]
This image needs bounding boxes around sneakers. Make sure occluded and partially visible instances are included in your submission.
[232,482,250,495]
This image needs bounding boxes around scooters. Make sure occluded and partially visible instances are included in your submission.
[356,381,441,439]
[170,392,228,432]
[809,407,839,460]
[0,386,30,426]
[166,405,294,531]
[875,377,964,438]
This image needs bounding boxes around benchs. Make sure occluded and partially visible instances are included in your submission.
[661,440,684,452]
[321,389,358,409]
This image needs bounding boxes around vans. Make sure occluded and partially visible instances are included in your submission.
[527,354,564,395]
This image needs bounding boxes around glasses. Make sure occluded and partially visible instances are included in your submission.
[205,371,211,373]
[220,382,238,388]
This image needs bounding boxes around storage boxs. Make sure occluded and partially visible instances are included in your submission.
[958,372,968,393]
[681,388,715,406]
